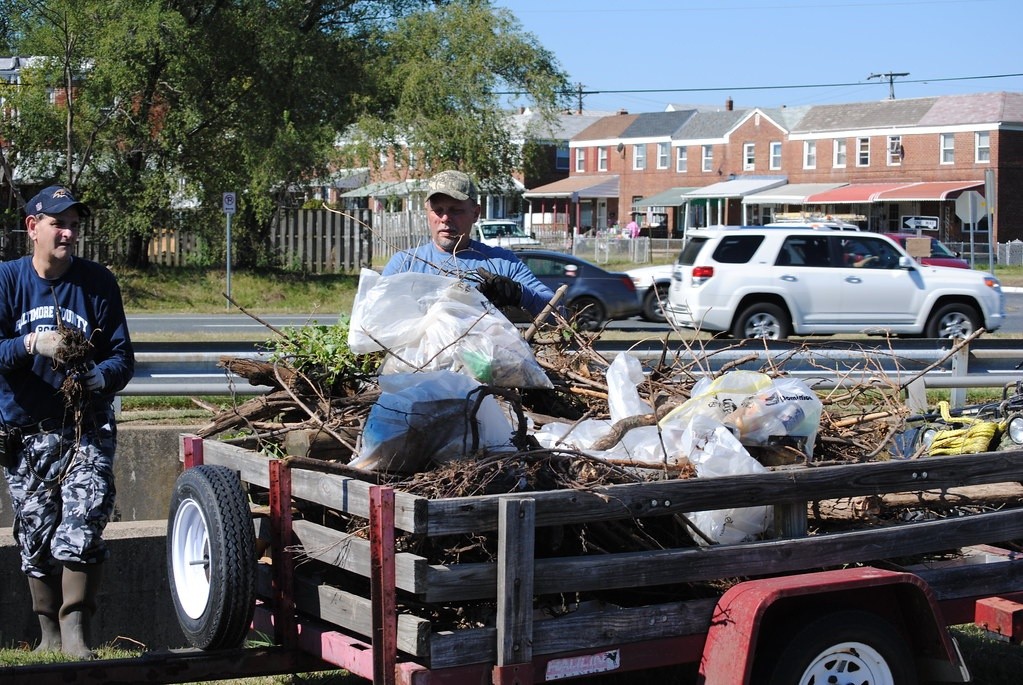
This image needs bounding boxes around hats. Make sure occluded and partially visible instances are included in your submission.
[24,184,91,218]
[425,169,477,206]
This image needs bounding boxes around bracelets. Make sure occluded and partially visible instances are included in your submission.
[27,332,32,352]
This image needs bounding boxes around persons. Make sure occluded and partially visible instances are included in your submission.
[383,169,565,324]
[0,185,134,660]
[845,244,879,268]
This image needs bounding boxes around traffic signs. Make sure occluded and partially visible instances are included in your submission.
[900,216,940,232]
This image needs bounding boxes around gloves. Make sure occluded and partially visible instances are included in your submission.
[30,330,77,364]
[65,359,105,394]
[474,267,522,308]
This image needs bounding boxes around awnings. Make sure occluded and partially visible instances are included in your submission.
[875,180,986,202]
[523,175,619,235]
[806,183,912,230]
[629,188,705,236]
[742,182,849,226]
[681,176,787,250]
[300,168,370,187]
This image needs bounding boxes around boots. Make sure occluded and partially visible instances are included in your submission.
[58,561,99,659]
[27,575,62,654]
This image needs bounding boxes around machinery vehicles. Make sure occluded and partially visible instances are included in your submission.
[161,375,1022,685]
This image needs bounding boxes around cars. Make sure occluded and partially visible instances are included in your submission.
[880,231,971,269]
[623,261,675,324]
[499,250,646,332]
[471,218,544,251]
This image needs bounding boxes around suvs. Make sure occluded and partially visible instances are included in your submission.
[662,224,1008,340]
[760,210,869,248]
